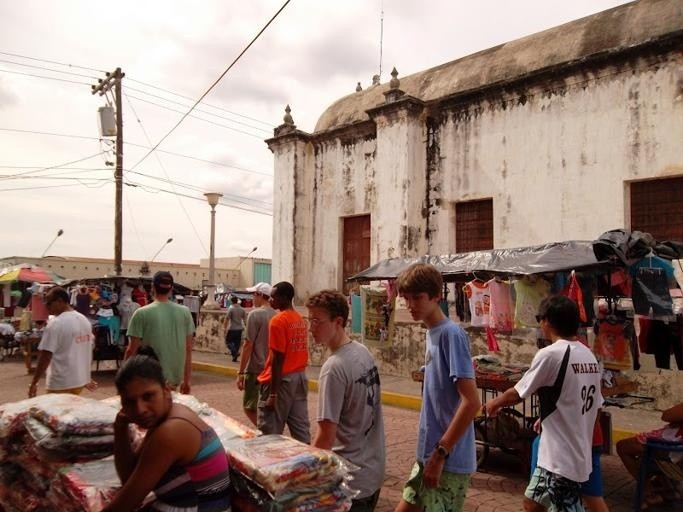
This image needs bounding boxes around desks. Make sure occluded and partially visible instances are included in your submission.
[18,322,124,373]
[412,361,638,480]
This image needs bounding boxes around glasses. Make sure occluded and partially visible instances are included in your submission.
[301,316,322,326]
[534,313,546,324]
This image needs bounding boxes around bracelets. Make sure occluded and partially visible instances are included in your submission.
[236,370,245,374]
[28,382,42,388]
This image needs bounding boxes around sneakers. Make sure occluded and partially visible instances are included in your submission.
[643,495,663,508]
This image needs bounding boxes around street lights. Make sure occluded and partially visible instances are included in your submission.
[202,190,222,337]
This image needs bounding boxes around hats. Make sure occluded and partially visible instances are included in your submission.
[153,271,174,289]
[246,282,272,296]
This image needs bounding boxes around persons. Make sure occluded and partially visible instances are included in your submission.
[124,270,195,394]
[304,288,384,512]
[220,296,247,362]
[530,416,610,512]
[256,282,314,446]
[392,263,481,512]
[96,345,234,512]
[482,295,605,512]
[233,281,278,425]
[26,286,98,395]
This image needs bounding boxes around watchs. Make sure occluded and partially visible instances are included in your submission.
[432,442,450,460]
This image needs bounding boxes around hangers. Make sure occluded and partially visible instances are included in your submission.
[71,279,88,290]
[372,278,389,290]
[440,245,680,326]
[352,279,361,289]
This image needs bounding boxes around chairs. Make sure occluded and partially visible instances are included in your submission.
[632,440,683,512]
